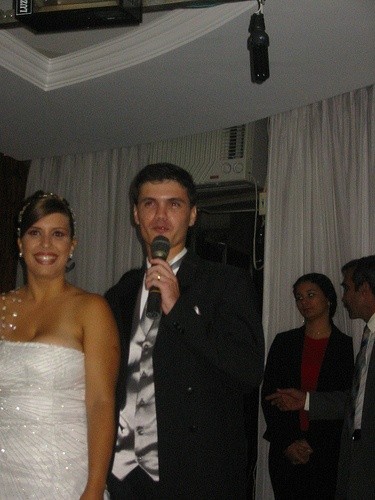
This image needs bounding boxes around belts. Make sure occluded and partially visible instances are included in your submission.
[352,430,361,440]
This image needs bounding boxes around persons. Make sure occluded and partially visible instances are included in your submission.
[261,254,375,500]
[0,189,121,500]
[102,163,265,500]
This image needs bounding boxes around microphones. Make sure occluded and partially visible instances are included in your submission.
[146,236,170,318]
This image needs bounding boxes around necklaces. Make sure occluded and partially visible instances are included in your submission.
[306,330,330,335]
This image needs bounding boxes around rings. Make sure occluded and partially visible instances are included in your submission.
[158,273,161,281]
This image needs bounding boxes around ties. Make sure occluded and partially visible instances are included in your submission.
[348,325,370,436]
